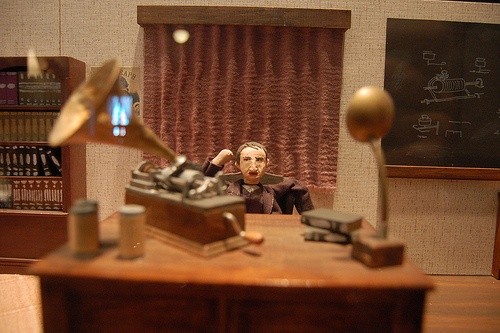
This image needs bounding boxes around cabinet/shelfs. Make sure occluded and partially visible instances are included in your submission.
[0,56,87,274]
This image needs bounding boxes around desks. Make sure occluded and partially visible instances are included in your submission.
[26,210,435,333]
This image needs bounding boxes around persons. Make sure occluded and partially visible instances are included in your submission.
[201,142,315,215]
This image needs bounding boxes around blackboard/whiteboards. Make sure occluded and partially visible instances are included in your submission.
[381,17,500,181]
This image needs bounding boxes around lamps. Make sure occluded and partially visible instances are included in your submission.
[344,85,409,268]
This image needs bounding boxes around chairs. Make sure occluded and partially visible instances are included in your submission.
[220,170,284,191]
